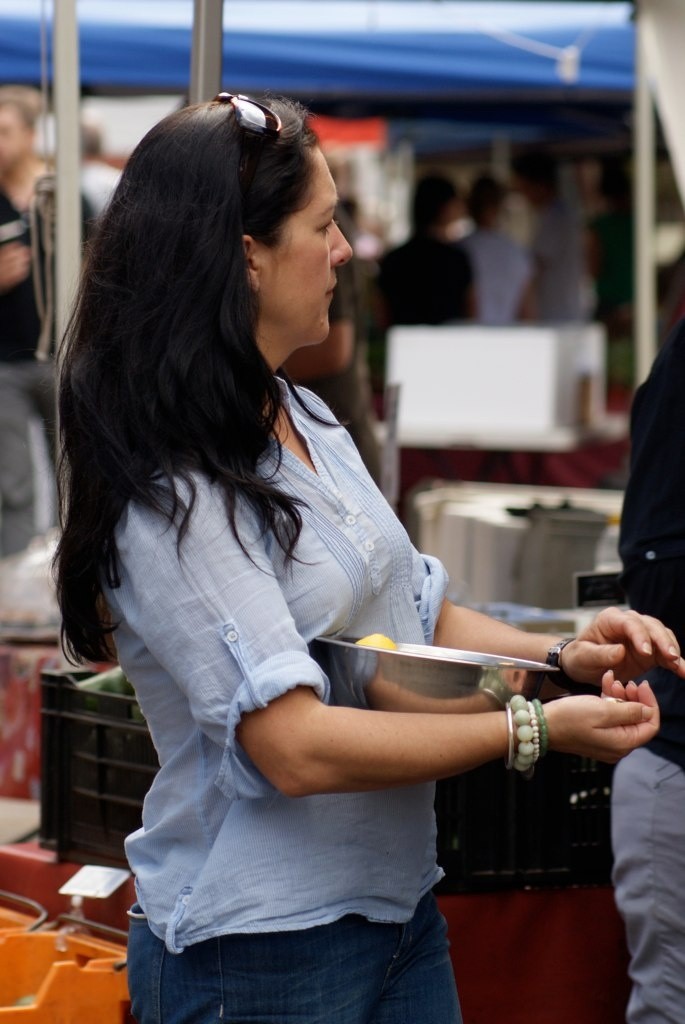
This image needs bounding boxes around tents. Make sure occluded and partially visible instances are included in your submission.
[1,0,631,531]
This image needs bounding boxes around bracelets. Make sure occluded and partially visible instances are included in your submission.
[480,674,515,708]
[545,638,603,696]
[504,694,548,781]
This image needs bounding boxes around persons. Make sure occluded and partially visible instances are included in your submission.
[309,638,527,716]
[0,84,633,555]
[612,313,684,1024]
[53,91,685,1024]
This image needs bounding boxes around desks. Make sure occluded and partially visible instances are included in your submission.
[0,840,634,1024]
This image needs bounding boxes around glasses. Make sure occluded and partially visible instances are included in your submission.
[217,90,286,227]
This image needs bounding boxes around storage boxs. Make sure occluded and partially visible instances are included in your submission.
[386,324,608,452]
[40,667,615,891]
[414,483,627,605]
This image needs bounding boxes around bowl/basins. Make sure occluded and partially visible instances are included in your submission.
[310,635,560,714]
